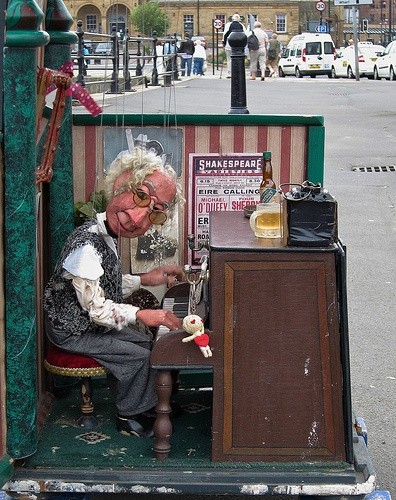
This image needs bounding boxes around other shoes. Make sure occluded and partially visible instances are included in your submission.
[249,70,277,82]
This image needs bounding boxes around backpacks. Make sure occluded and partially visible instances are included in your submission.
[248,31,259,51]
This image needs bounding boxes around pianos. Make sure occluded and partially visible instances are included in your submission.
[150,208,353,463]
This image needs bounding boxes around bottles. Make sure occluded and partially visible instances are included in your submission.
[260,151,277,202]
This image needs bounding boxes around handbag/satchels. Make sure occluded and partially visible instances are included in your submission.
[268,49,277,59]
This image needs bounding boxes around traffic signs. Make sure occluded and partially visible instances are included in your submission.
[315,25,326,32]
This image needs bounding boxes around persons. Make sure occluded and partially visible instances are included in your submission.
[84,46,89,65]
[265,33,281,77]
[247,21,270,81]
[334,35,391,49]
[178,32,195,76]
[192,40,207,76]
[40,145,188,436]
[220,13,247,78]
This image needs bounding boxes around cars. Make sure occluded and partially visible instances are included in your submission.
[69,38,92,56]
[331,41,385,79]
[373,40,396,81]
[94,41,115,56]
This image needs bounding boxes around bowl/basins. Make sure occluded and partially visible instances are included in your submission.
[243,205,258,218]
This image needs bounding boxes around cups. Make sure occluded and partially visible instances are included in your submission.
[250,203,284,239]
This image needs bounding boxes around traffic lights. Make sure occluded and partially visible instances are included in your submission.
[362,20,368,32]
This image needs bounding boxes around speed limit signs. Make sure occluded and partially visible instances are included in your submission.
[214,19,223,30]
[315,1,327,12]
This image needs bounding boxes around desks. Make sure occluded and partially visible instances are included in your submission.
[207,210,347,466]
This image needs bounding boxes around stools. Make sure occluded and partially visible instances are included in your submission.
[44,348,112,429]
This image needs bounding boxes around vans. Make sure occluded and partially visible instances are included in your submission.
[277,33,336,78]
